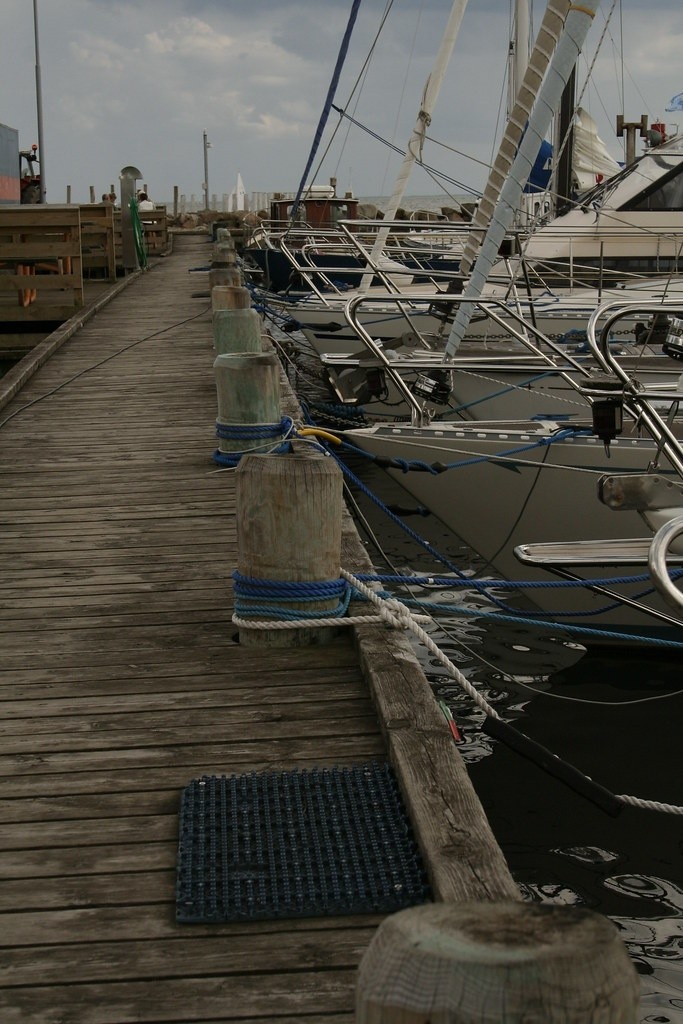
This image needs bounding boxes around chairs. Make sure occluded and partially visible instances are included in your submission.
[144,220,156,250]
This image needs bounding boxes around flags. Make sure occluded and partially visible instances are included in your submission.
[665,92,683,112]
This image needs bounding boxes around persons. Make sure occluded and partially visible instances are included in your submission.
[101,190,158,238]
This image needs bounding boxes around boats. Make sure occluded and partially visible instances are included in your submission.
[240,1,683,635]
